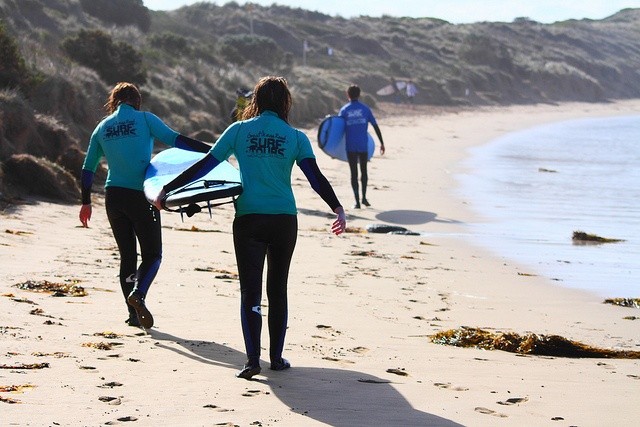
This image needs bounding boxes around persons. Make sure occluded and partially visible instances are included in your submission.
[78,82,228,329]
[155,74,346,378]
[406,80,420,109]
[338,85,385,209]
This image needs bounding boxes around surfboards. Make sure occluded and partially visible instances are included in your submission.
[144,145,244,221]
[317,115,375,163]
